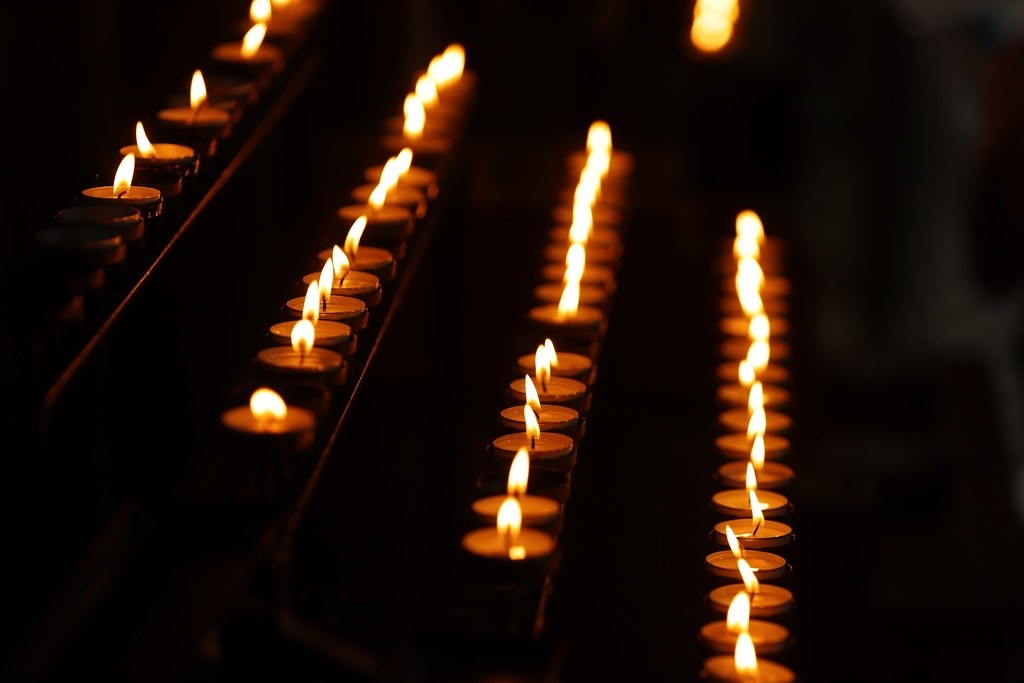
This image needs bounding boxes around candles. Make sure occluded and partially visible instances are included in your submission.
[84,25,794,683]
